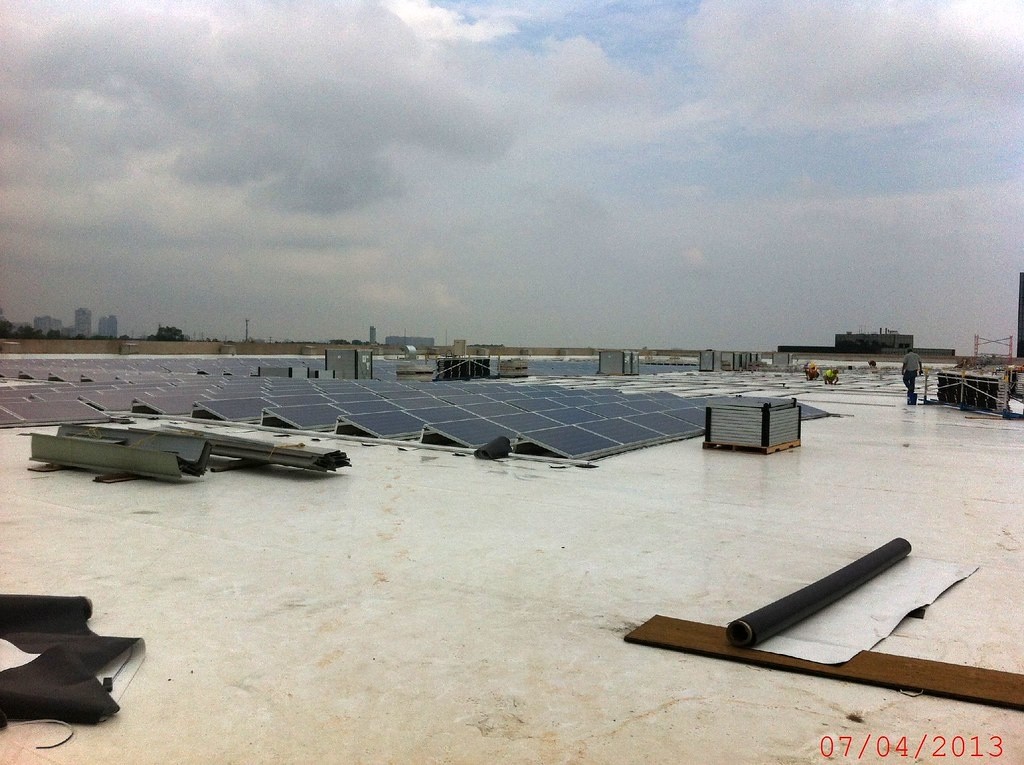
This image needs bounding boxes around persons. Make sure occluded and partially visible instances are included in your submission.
[902,348,923,397]
[823,369,839,385]
[803,361,820,381]
